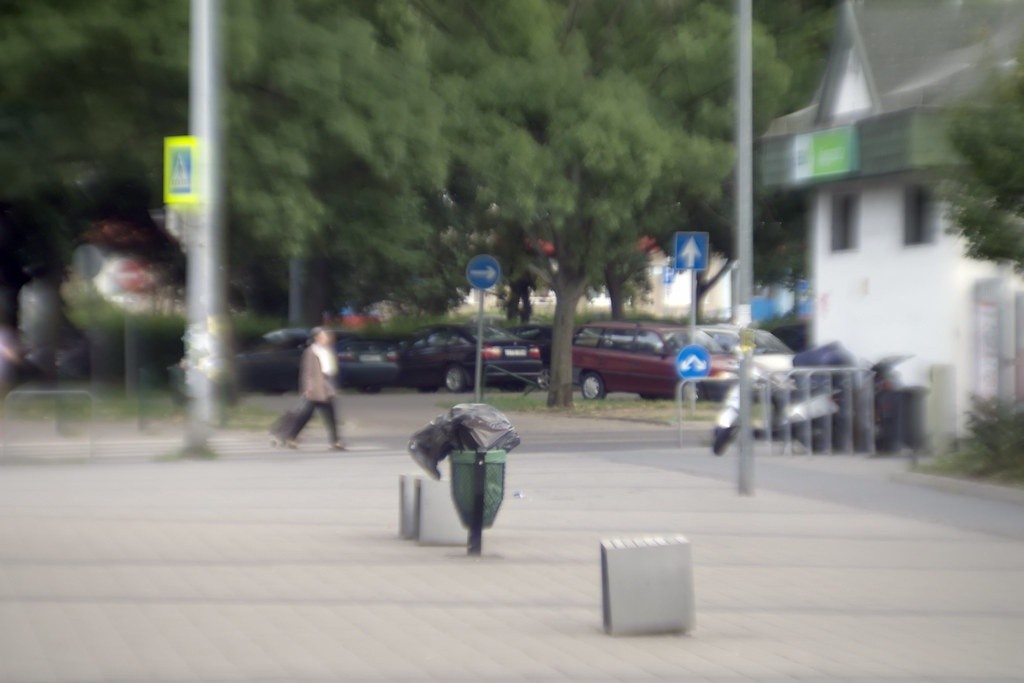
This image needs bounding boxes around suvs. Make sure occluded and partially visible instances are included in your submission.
[568,322,763,400]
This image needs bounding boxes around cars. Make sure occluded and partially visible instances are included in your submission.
[659,326,797,373]
[509,325,614,390]
[393,325,543,394]
[231,328,400,394]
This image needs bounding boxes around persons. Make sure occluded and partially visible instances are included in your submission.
[284,326,344,451]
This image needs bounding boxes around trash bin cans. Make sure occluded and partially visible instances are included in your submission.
[452,448,506,529]
